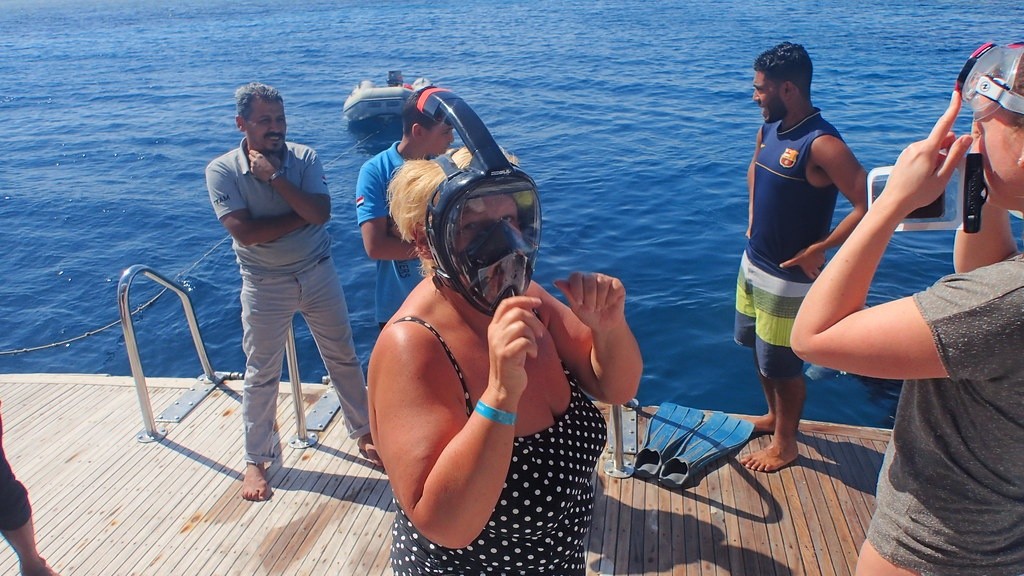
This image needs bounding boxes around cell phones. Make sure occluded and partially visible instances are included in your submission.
[872,180,945,218]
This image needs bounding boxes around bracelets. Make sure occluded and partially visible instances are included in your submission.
[473,400,517,426]
[266,169,280,186]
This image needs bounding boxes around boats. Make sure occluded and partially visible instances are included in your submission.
[343,70,431,120]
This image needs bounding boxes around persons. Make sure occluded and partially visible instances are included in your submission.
[205,83,387,503]
[788,34,1023,576]
[364,147,642,576]
[0,401,64,576]
[354,109,460,330]
[733,40,870,473]
